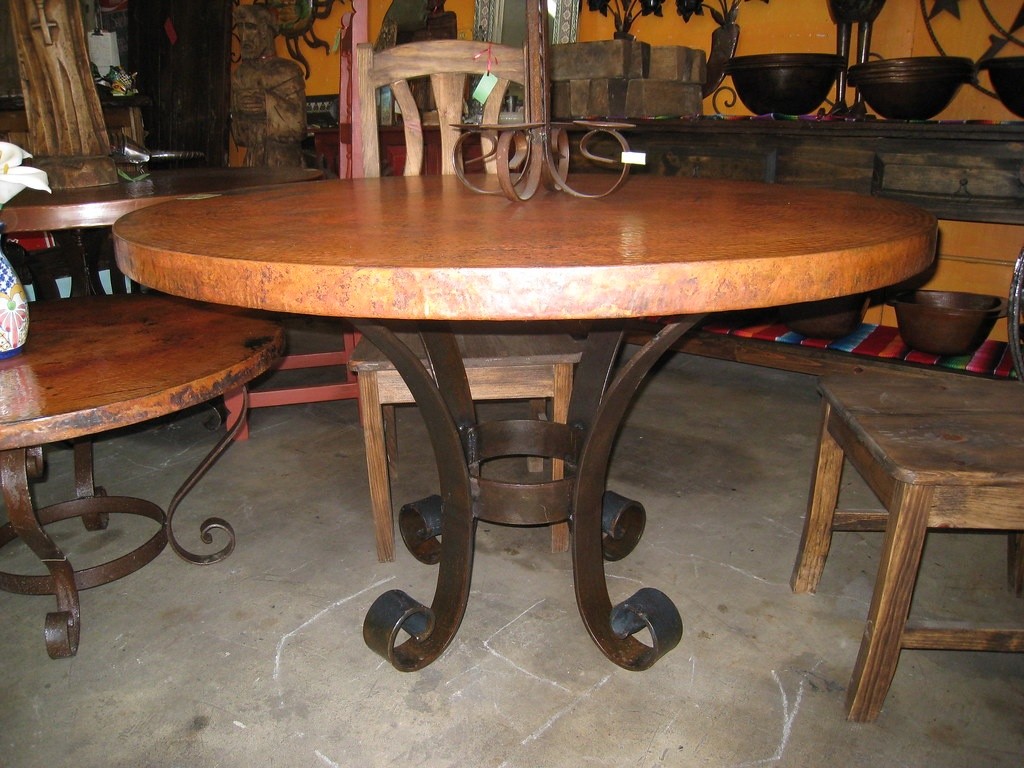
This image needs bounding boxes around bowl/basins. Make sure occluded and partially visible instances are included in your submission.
[978,55,1024,119]
[847,55,980,120]
[885,289,1008,356]
[780,290,880,339]
[720,53,847,116]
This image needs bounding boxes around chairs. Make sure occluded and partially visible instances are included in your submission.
[790,370,1024,723]
[347,38,583,563]
[225,0,368,440]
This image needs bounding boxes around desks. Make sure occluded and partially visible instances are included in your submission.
[0,166,325,484]
[0,90,154,157]
[113,173,938,672]
[0,292,286,660]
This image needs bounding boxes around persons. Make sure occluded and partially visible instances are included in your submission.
[232,4,309,169]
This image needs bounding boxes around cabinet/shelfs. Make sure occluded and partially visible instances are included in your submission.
[566,118,1024,377]
[302,124,524,182]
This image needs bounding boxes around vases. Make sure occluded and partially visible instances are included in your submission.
[0,222,30,358]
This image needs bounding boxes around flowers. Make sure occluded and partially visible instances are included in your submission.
[585,0,769,33]
[0,142,52,211]
